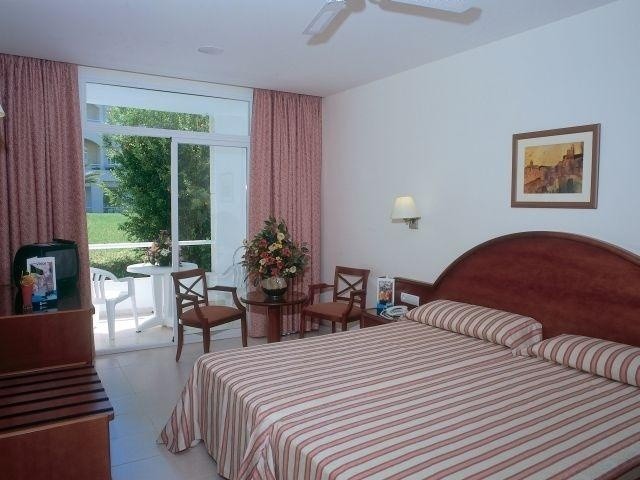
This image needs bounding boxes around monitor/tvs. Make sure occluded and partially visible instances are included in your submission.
[11,243,82,300]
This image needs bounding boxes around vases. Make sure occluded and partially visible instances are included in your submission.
[260,277,287,297]
[159,258,169,266]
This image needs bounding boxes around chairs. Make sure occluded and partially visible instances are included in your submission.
[300,266,371,336]
[90,266,138,340]
[171,269,247,361]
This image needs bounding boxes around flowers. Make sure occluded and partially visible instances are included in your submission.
[237,215,309,281]
[144,230,182,266]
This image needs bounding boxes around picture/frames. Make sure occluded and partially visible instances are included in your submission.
[26,256,56,303]
[377,278,396,306]
[511,123,601,209]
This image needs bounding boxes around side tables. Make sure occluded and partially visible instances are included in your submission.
[240,290,307,343]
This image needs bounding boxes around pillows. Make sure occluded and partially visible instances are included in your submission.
[516,333,640,387]
[405,299,542,355]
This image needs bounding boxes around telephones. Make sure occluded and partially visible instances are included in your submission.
[380,305,408,320]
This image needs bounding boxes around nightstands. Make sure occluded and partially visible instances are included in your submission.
[360,308,396,328]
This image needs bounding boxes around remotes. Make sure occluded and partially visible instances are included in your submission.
[51,238,75,245]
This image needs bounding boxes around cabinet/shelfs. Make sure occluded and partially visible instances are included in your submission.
[0,367,115,480]
[0,281,95,376]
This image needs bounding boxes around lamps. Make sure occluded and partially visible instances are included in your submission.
[390,196,421,230]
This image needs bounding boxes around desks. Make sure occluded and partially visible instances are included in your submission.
[127,262,198,332]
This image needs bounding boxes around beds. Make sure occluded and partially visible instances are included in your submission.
[156,230,640,480]
